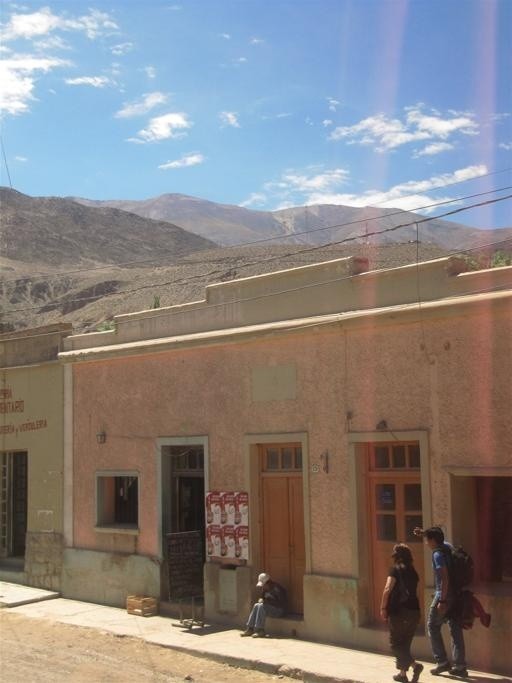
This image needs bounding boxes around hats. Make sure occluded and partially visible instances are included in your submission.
[255,573,271,587]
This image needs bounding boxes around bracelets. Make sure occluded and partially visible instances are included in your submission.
[437,598,447,604]
[381,607,386,610]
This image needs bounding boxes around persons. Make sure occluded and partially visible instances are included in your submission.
[239,572,289,639]
[379,542,424,683]
[412,525,471,678]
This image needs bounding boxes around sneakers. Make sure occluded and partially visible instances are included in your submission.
[430,661,452,675]
[411,664,424,682]
[449,667,469,677]
[240,629,265,637]
[393,674,409,682]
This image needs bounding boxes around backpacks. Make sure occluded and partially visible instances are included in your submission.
[432,544,474,590]
